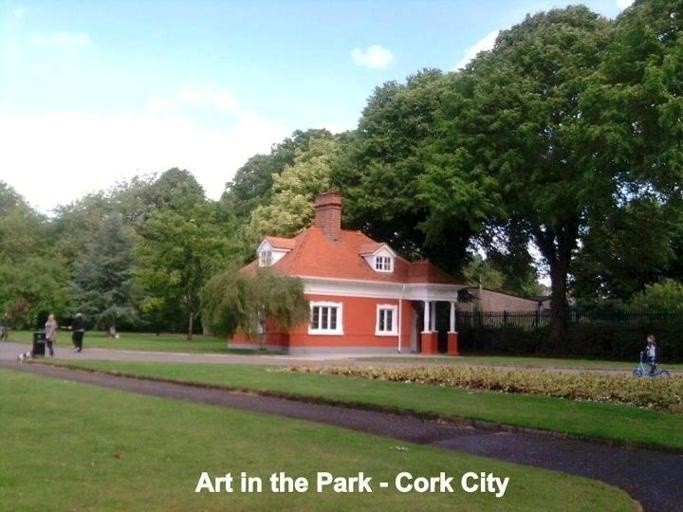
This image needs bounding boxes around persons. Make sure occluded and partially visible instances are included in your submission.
[45,314,57,357]
[0,312,12,342]
[641,335,656,375]
[68,313,84,352]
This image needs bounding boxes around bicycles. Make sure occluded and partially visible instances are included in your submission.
[634,351,671,378]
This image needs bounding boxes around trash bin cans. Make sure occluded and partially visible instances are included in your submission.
[32,332,45,355]
